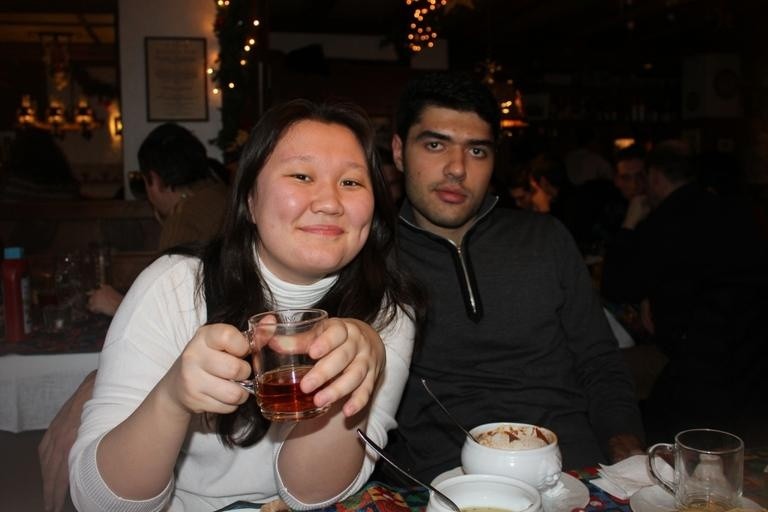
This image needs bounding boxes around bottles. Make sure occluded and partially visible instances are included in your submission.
[3,247,35,342]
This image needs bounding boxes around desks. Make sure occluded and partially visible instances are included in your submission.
[1,317,112,438]
[218,426,768,509]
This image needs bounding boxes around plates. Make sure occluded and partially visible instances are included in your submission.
[428,466,590,512]
[630,481,766,511]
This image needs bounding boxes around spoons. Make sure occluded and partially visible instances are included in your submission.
[357,428,460,512]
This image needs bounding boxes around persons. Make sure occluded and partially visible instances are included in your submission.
[376,141,768,450]
[60,99,429,512]
[38,86,654,512]
[84,124,233,319]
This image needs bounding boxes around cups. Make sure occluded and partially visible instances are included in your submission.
[645,429,744,511]
[229,309,331,421]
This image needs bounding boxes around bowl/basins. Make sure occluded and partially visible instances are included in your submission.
[461,422,564,495]
[425,475,544,512]
